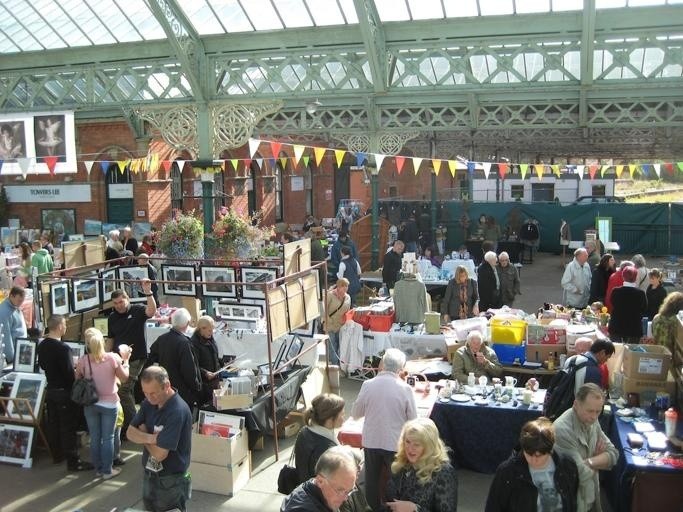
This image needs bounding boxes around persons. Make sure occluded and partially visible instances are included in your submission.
[37,118,64,155]
[0,124,23,159]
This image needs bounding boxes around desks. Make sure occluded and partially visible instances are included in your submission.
[606,399,682,512]
[431,380,546,481]
[333,376,445,458]
[360,272,451,311]
[567,241,621,259]
[465,236,522,270]
[145,322,327,450]
[487,361,561,388]
[342,321,450,381]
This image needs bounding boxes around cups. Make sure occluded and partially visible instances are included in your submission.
[118,344,127,353]
[406,376,414,386]
[628,392,639,407]
[468,371,517,405]
[522,390,532,405]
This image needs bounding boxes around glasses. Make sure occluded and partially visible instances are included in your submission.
[319,475,358,499]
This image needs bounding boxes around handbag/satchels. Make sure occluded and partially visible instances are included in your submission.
[277,447,299,495]
[70,354,98,406]
[144,480,187,512]
[317,323,323,334]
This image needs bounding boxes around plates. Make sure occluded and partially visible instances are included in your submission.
[450,393,469,402]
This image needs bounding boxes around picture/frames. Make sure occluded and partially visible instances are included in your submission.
[143,298,266,335]
[1,423,35,469]
[2,337,86,389]
[266,238,320,342]
[120,265,150,303]
[92,317,109,337]
[50,280,69,316]
[2,205,326,292]
[200,266,237,298]
[73,274,101,312]
[101,266,120,303]
[161,264,197,297]
[2,373,47,423]
[241,265,278,299]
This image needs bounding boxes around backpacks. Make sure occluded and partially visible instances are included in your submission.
[542,356,595,422]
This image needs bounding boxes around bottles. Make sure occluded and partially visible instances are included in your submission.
[512,357,521,367]
[665,407,677,436]
[642,317,647,336]
[444,249,469,261]
[647,322,652,337]
[377,283,388,297]
[401,256,435,282]
[547,352,554,370]
[558,353,566,367]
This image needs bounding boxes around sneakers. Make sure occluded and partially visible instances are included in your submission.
[68,458,125,480]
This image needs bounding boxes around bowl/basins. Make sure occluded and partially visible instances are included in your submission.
[522,364,541,369]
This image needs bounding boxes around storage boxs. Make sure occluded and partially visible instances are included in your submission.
[367,306,395,331]
[669,311,682,370]
[564,331,602,356]
[619,372,678,406]
[344,308,370,331]
[525,342,565,365]
[621,342,670,380]
[184,451,252,498]
[490,318,525,344]
[492,340,525,366]
[190,424,250,467]
